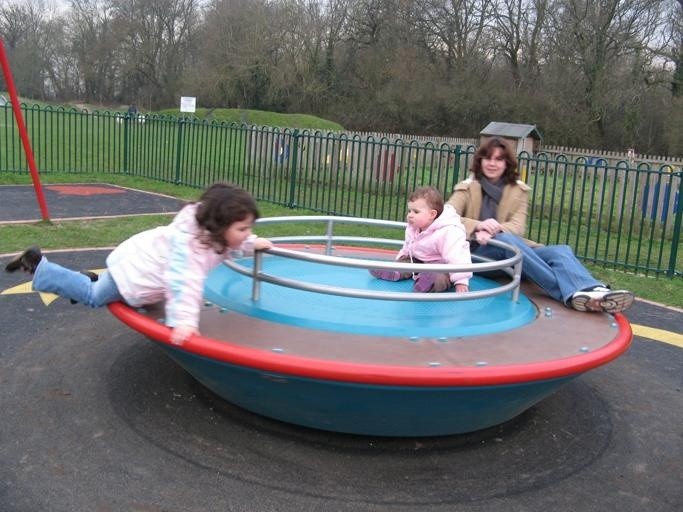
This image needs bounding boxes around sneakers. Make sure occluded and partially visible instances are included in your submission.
[369,268,401,282]
[570,286,634,314]
[413,271,436,293]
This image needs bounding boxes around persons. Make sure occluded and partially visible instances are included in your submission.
[366,186,473,294]
[5,184,273,347]
[444,138,634,315]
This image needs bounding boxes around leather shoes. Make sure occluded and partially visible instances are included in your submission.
[68,266,99,304]
[6,246,42,274]
